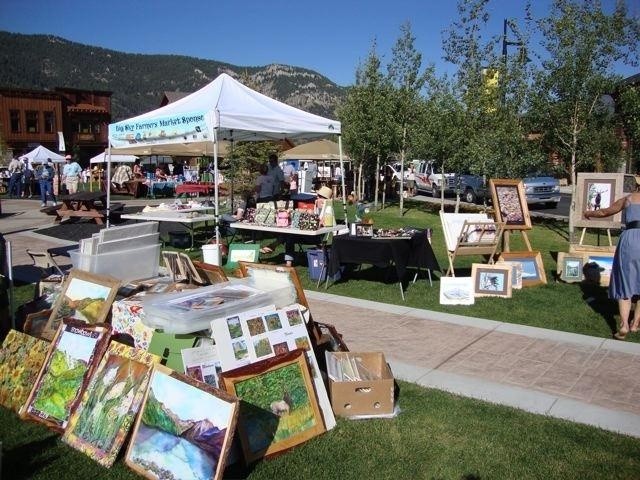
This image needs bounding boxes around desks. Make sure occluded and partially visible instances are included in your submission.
[230,214,347,274]
[326,222,434,301]
[118,210,223,249]
[155,207,215,234]
[55,190,112,226]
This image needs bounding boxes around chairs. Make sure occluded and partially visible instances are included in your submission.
[26,249,73,301]
[0,233,49,330]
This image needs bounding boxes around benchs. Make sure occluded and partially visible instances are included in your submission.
[40,205,76,219]
[56,209,104,226]
[99,203,126,223]
[95,201,120,216]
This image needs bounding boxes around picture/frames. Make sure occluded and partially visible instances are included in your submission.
[472,263,512,298]
[24,308,53,339]
[20,323,110,435]
[60,340,161,470]
[228,243,260,263]
[576,172,623,230]
[438,276,474,304]
[583,251,616,287]
[193,260,226,284]
[490,179,533,231]
[42,266,120,341]
[496,260,523,290]
[501,250,547,287]
[221,349,327,467]
[126,362,240,480]
[1,329,51,415]
[238,261,309,310]
[556,251,585,285]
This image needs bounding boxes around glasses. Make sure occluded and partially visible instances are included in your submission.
[66,159,71,160]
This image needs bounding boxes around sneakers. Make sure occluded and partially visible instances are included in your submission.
[42,203,46,207]
[52,202,57,206]
[27,194,33,200]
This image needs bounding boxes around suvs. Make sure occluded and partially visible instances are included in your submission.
[394,159,425,187]
[415,159,454,198]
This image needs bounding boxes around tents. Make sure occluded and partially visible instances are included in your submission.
[106,71,348,246]
[89,150,140,192]
[276,138,352,179]
[104,138,242,164]
[18,145,66,167]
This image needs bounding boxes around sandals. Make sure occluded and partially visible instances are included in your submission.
[259,246,274,255]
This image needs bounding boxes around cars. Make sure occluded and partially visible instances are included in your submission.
[380,163,399,189]
[454,172,562,211]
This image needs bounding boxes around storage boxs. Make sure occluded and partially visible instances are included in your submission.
[305,245,343,281]
[67,242,163,280]
[323,349,396,417]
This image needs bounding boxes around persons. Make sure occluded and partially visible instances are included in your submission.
[22,157,35,199]
[255,164,275,202]
[155,168,167,181]
[35,161,57,207]
[7,156,21,199]
[585,174,640,340]
[269,155,284,197]
[61,155,82,195]
[133,159,142,179]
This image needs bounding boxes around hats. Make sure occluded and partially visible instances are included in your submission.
[65,154,72,161]
[633,173,640,185]
[316,186,333,200]
[22,157,27,161]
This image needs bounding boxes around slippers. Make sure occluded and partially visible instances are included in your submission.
[613,330,628,341]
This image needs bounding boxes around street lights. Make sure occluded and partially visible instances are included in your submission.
[499,18,532,104]
